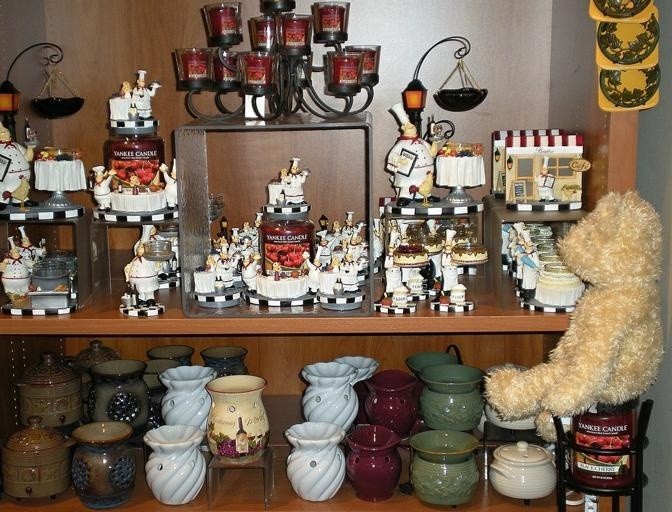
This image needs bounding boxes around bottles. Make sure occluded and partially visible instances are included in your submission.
[1,340,559,509]
[22,115,31,144]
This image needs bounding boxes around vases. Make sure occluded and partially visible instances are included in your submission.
[344,426,401,502]
[147,345,196,365]
[159,366,217,431]
[335,355,378,379]
[363,369,417,431]
[144,425,208,504]
[203,374,270,462]
[71,421,136,508]
[89,358,150,429]
[301,361,359,431]
[409,431,481,507]
[203,345,251,376]
[76,341,119,416]
[419,363,484,434]
[284,422,346,500]
[404,352,457,374]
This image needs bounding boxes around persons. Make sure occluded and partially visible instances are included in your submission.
[0,125,39,207]
[0,226,46,305]
[120,70,158,120]
[387,103,441,208]
[207,211,458,296]
[92,159,177,211]
[124,225,171,305]
[282,157,309,206]
[502,222,539,301]
[0,62,591,315]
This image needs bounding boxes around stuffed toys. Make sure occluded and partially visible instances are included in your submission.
[480,189,670,443]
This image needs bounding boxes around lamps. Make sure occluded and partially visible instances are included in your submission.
[387,36,490,145]
[1,38,84,141]
[163,0,380,121]
